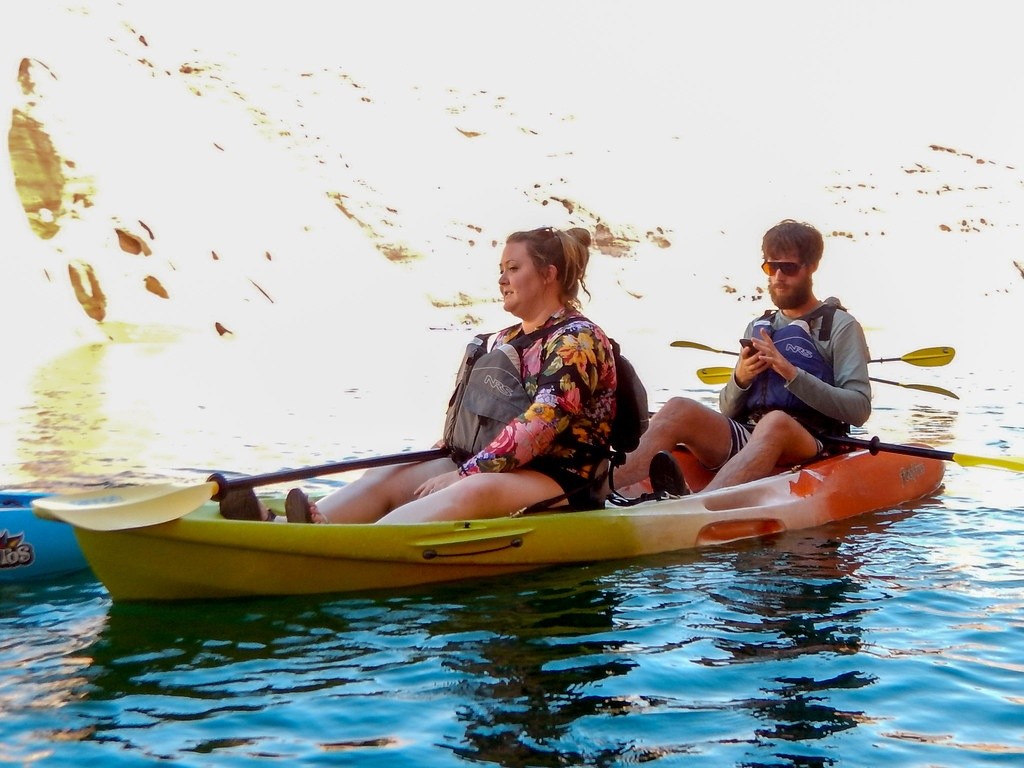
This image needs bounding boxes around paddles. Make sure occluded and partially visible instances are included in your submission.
[31,447,447,533]
[696,346,955,385]
[670,340,958,400]
[649,411,1024,473]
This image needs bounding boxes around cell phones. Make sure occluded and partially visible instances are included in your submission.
[740,338,758,357]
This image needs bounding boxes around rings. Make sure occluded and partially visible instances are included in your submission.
[429,489,436,494]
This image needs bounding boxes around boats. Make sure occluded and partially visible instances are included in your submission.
[32,440,945,601]
[1,488,327,594]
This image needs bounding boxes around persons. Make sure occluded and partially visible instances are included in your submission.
[218,227,617,523]
[597,219,872,504]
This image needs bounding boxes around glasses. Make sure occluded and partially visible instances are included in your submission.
[760,259,807,276]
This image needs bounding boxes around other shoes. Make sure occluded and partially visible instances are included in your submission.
[286,488,328,524]
[220,485,276,522]
[648,450,693,499]
